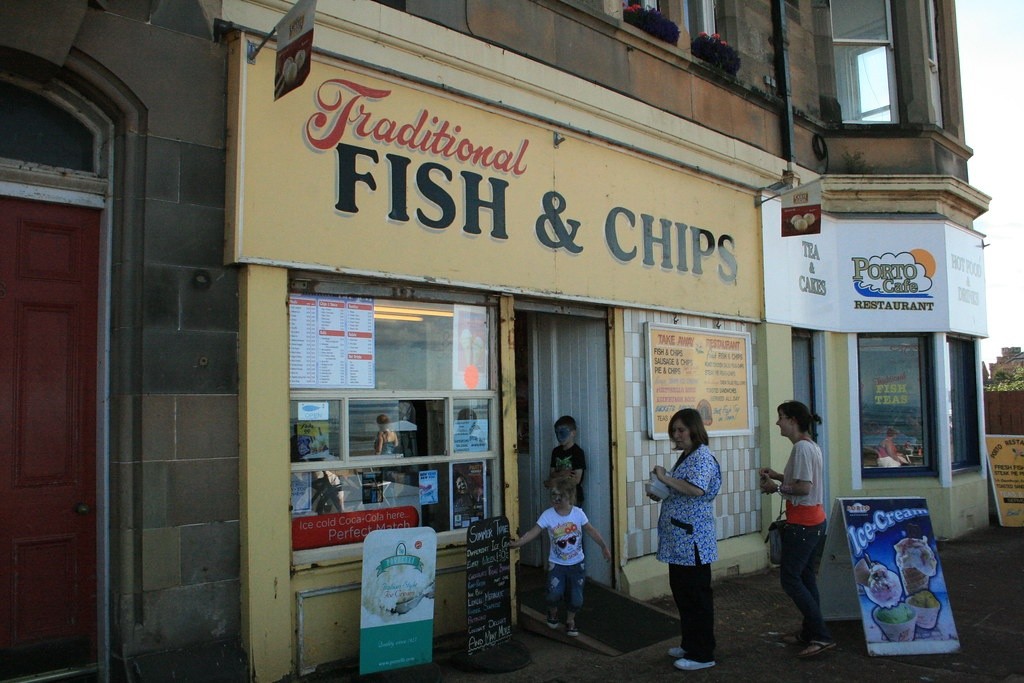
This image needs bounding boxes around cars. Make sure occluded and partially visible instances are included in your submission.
[862,432,923,465]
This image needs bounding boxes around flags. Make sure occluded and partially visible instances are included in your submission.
[779,176,822,238]
[272,1,315,102]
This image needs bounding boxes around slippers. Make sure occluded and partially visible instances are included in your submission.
[783,631,836,657]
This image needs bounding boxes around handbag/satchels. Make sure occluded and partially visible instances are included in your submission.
[769,519,788,564]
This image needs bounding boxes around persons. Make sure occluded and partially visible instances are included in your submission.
[373,414,399,456]
[753,399,836,659]
[549,415,587,510]
[453,408,487,454]
[641,408,722,673]
[293,450,348,516]
[453,472,475,528]
[875,428,907,467]
[510,468,612,637]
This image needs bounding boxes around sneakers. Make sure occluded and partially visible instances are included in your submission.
[668,646,715,669]
[547,605,578,637]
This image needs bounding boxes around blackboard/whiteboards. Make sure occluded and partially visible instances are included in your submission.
[463,515,514,656]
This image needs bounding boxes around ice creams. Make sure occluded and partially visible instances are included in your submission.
[854,523,941,624]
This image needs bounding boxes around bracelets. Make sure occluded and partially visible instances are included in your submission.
[777,484,784,498]
